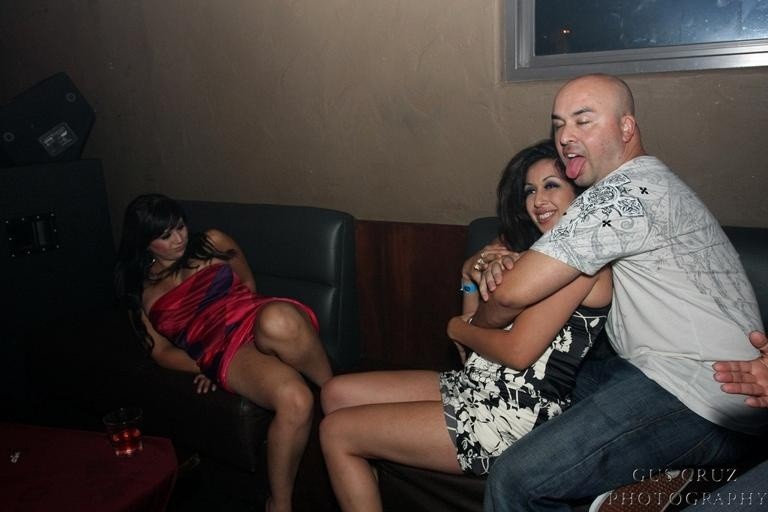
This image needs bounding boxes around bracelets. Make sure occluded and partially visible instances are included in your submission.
[459,280,479,294]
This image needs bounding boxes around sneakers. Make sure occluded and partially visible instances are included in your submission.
[588,466,697,512]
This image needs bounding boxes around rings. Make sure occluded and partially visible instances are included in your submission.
[475,257,485,264]
[480,250,488,258]
[473,263,480,272]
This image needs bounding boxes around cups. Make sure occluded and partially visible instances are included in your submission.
[102,404,144,460]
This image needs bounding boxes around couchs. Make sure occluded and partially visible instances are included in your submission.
[122,191,767,511]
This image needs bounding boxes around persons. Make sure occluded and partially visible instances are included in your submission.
[711,329,768,409]
[468,73,767,512]
[113,193,332,511]
[319,136,616,512]
